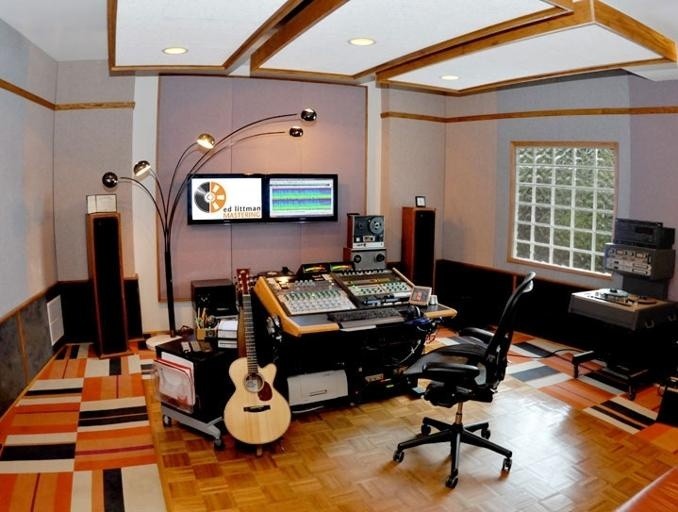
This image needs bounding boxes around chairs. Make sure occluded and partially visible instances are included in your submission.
[392,271,537,488]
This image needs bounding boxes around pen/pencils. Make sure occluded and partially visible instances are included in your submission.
[197,307,207,319]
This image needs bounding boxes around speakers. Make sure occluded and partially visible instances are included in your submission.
[192,279,236,313]
[86,212,136,359]
[400,206,436,295]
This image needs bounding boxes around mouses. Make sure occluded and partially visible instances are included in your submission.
[407,305,420,318]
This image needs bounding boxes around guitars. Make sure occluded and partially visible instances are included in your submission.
[222,268,292,444]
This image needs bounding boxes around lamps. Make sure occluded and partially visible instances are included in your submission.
[103,108,317,337]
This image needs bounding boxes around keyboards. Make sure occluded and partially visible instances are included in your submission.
[328,308,405,328]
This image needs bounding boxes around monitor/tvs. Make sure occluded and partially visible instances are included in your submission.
[187,173,265,225]
[265,174,338,222]
